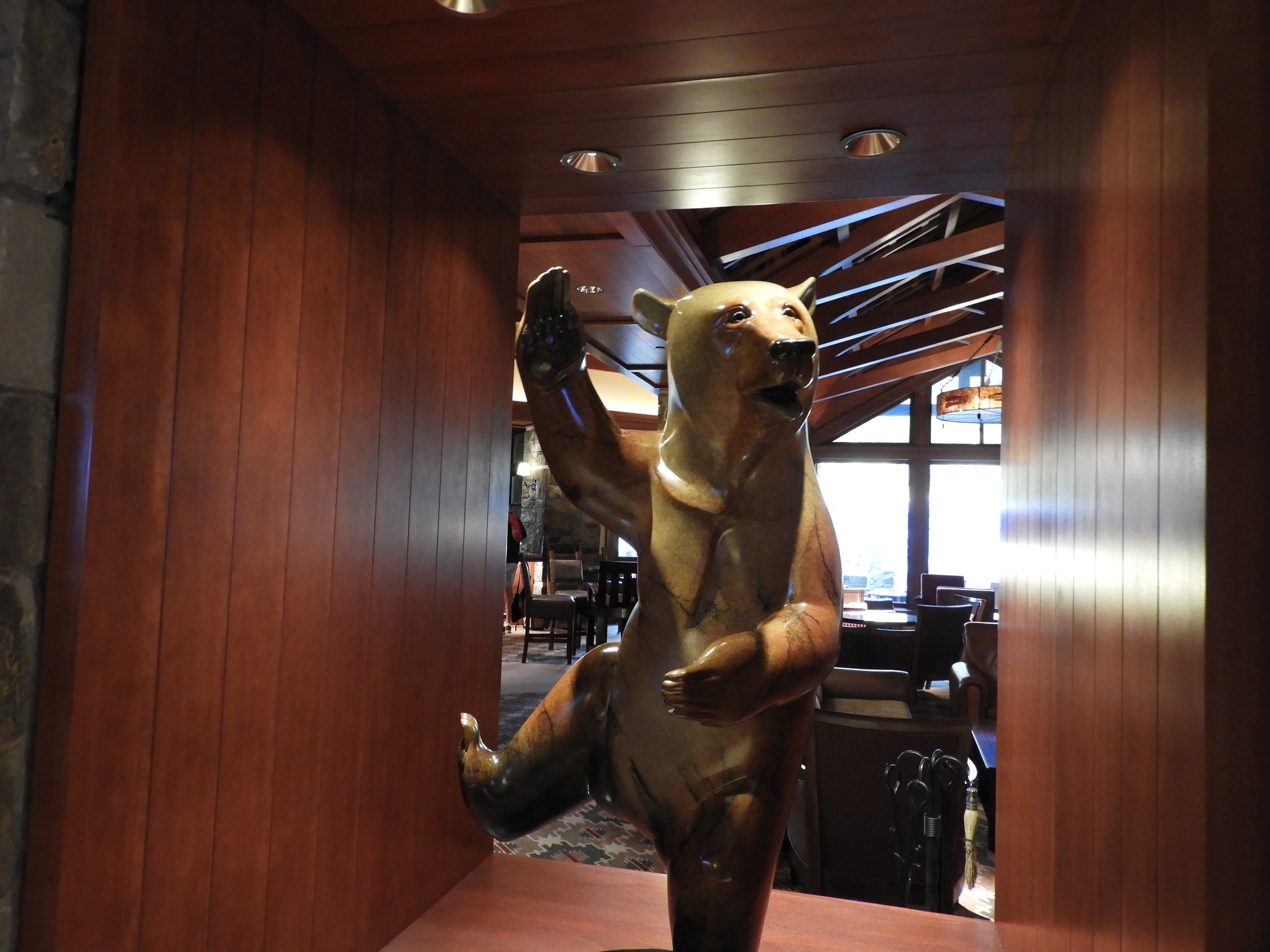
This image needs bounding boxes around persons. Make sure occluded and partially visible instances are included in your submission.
[501,510,528,634]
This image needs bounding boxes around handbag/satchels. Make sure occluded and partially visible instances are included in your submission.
[510,586,528,622]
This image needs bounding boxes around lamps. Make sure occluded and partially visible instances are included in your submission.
[516,462,550,476]
[936,339,1002,423]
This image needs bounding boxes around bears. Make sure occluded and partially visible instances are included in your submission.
[455,258,846,952]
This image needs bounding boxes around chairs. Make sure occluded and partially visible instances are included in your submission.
[785,573,1000,915]
[518,551,638,664]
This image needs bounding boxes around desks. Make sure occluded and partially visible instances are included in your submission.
[842,610,917,670]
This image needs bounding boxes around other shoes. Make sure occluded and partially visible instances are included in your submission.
[505,624,511,633]
[503,625,505,634]
